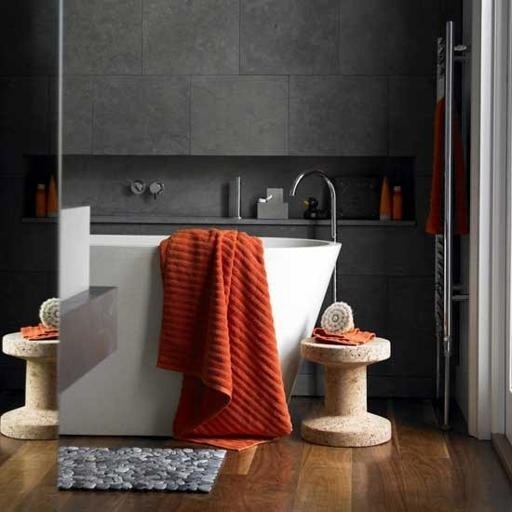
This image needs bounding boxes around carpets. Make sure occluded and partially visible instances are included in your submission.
[58,446,226,493]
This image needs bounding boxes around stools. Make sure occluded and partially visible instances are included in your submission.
[301,333,393,449]
[0,332,59,441]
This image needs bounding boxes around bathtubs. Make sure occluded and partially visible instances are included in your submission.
[90,228,341,436]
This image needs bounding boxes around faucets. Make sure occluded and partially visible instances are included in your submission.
[289,166,338,306]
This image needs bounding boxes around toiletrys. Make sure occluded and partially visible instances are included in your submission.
[379,178,391,219]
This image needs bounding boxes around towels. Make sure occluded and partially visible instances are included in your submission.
[157,229,293,452]
[312,328,376,345]
[21,324,58,341]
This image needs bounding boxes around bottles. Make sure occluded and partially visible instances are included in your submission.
[36,184,47,217]
